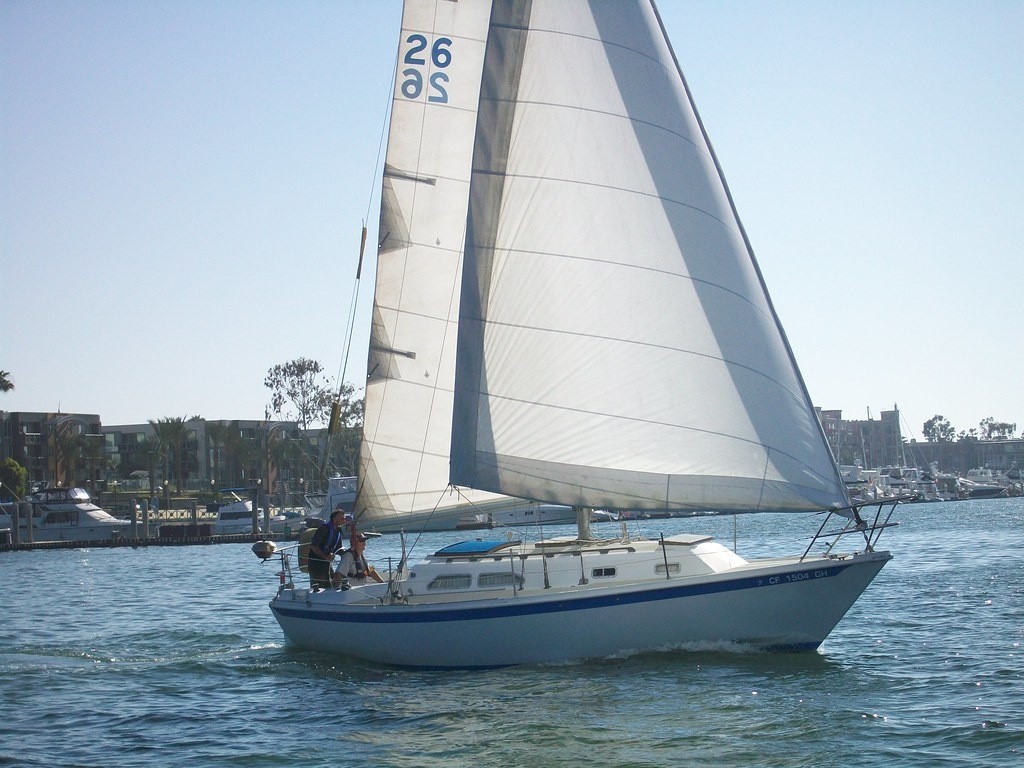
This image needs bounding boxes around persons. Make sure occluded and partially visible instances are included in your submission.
[308,509,346,589]
[333,533,385,588]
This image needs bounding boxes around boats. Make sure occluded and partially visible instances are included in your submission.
[212,499,307,537]
[486,503,620,523]
[0,486,159,543]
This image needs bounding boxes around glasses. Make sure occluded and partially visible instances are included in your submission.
[357,538,367,542]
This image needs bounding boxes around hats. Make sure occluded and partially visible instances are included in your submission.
[349,533,369,546]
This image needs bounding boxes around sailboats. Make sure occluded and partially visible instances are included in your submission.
[836,402,1022,500]
[251,3,898,674]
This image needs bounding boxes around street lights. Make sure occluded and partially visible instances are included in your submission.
[112,480,118,515]
[211,480,216,515]
[163,479,171,511]
[255,479,263,508]
[299,478,304,507]
